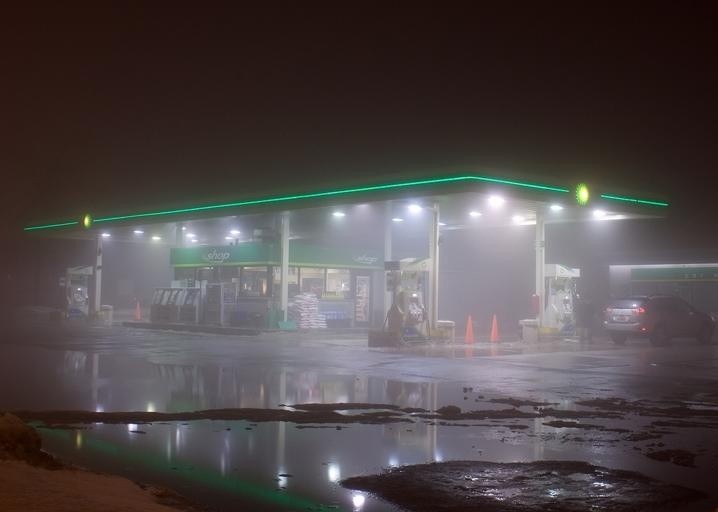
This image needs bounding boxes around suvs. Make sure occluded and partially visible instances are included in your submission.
[604,293,715,347]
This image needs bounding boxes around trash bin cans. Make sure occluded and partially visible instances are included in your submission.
[101,305,113,328]
[519,318,538,345]
[435,321,455,345]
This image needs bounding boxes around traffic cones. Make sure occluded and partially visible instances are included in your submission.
[489,315,501,343]
[135,302,142,321]
[463,315,476,345]
